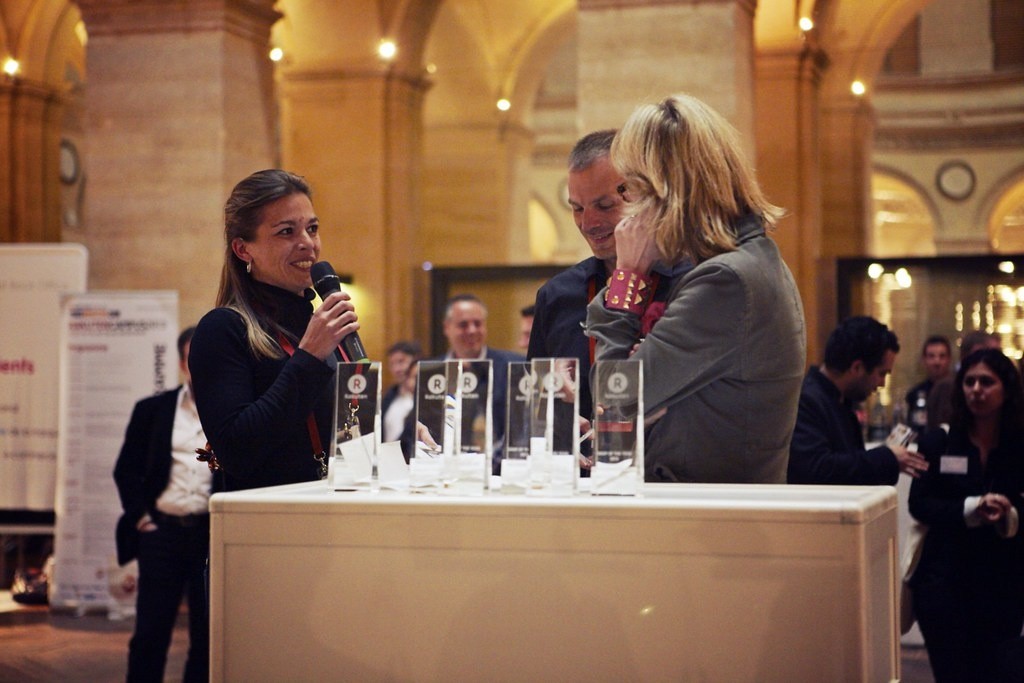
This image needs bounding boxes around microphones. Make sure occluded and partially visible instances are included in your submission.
[309,261,370,363]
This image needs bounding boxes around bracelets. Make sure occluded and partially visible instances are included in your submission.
[604,269,653,315]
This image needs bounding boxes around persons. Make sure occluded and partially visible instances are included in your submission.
[904,331,1024,683]
[789,315,930,487]
[189,170,384,496]
[382,294,530,475]
[520,305,537,350]
[113,326,211,683]
[524,130,694,478]
[579,94,806,482]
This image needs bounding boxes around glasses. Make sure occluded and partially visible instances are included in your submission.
[617,180,638,204]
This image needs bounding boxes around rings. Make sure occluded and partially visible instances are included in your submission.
[631,215,637,218]
[996,494,998,498]
